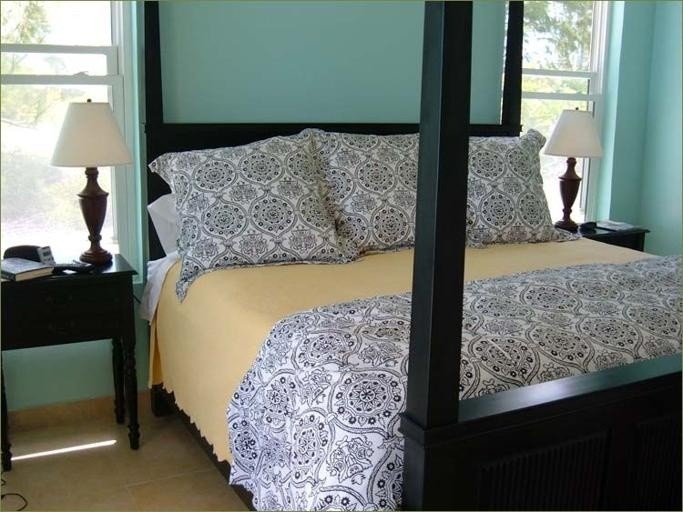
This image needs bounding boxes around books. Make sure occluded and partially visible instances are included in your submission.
[1,257,54,282]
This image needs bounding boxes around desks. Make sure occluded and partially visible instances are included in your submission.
[1,253,140,471]
[577,218,650,252]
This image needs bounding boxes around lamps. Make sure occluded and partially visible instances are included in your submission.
[543,107,603,234]
[51,98,135,265]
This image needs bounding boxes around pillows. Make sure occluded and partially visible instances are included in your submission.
[306,128,580,263]
[147,129,358,304]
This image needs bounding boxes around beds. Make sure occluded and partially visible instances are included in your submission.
[139,0,682,511]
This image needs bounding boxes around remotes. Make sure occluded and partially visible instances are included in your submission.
[51,263,94,274]
